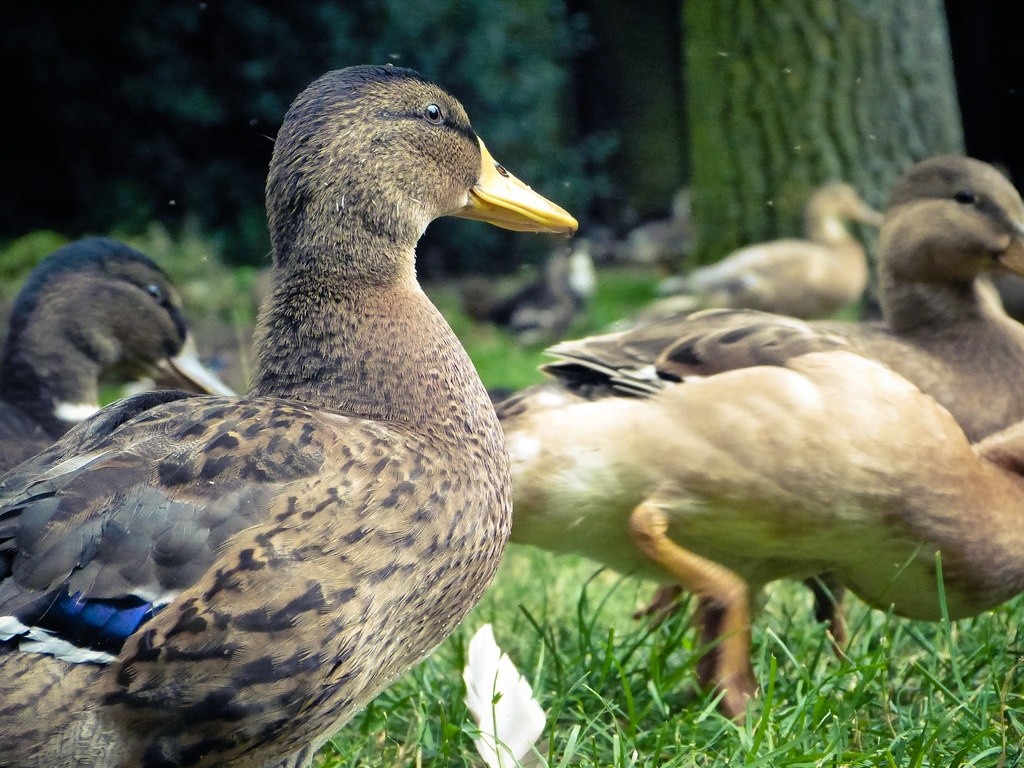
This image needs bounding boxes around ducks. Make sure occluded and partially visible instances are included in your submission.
[1,64,1024,768]
